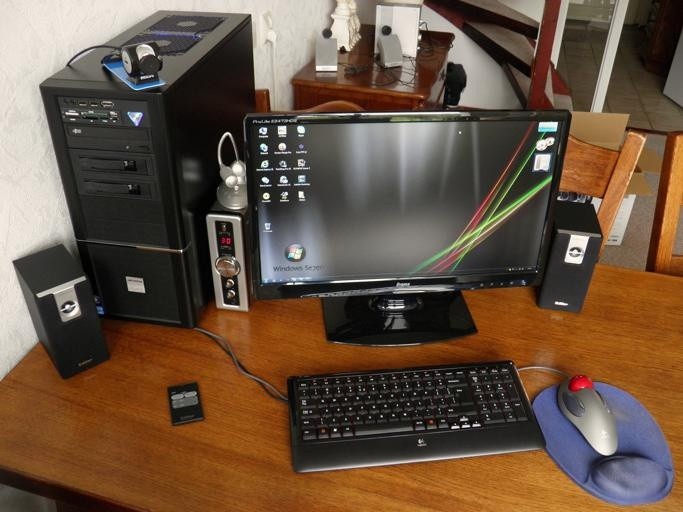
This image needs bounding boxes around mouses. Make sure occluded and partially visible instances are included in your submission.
[557,373,619,458]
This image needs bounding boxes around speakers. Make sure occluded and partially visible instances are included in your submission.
[12,244,112,379]
[315,38,337,72]
[377,34,403,68]
[535,192,604,314]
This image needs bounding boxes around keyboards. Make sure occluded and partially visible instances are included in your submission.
[287,359,547,474]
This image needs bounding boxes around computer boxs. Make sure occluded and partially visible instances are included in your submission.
[39,10,257,330]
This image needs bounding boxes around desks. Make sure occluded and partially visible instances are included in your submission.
[290,25,455,111]
[1,264,682,512]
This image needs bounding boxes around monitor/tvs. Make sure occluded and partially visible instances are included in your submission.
[240,108,571,346]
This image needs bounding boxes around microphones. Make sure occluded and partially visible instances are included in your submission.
[214,131,247,211]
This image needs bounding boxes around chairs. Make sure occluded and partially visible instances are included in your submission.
[559,131,682,273]
